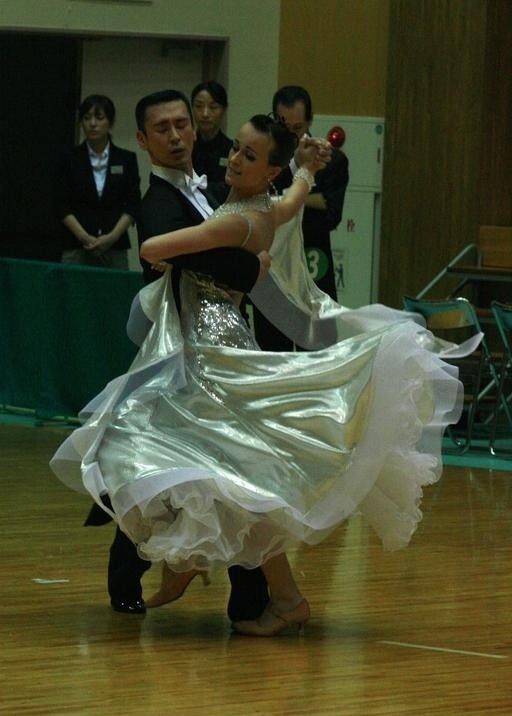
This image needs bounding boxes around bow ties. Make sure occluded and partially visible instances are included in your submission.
[185,173,207,193]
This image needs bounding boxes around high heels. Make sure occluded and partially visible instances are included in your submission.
[234,599,312,639]
[145,560,210,608]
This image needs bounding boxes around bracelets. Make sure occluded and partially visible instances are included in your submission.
[294,168,314,192]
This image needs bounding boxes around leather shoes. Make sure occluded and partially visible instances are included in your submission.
[111,597,146,613]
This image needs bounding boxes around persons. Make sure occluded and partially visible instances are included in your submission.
[53,94,142,418]
[50,113,485,636]
[84,90,333,619]
[240,86,349,352]
[191,83,233,211]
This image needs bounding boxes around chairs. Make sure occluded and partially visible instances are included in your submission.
[405,225,511,458]
